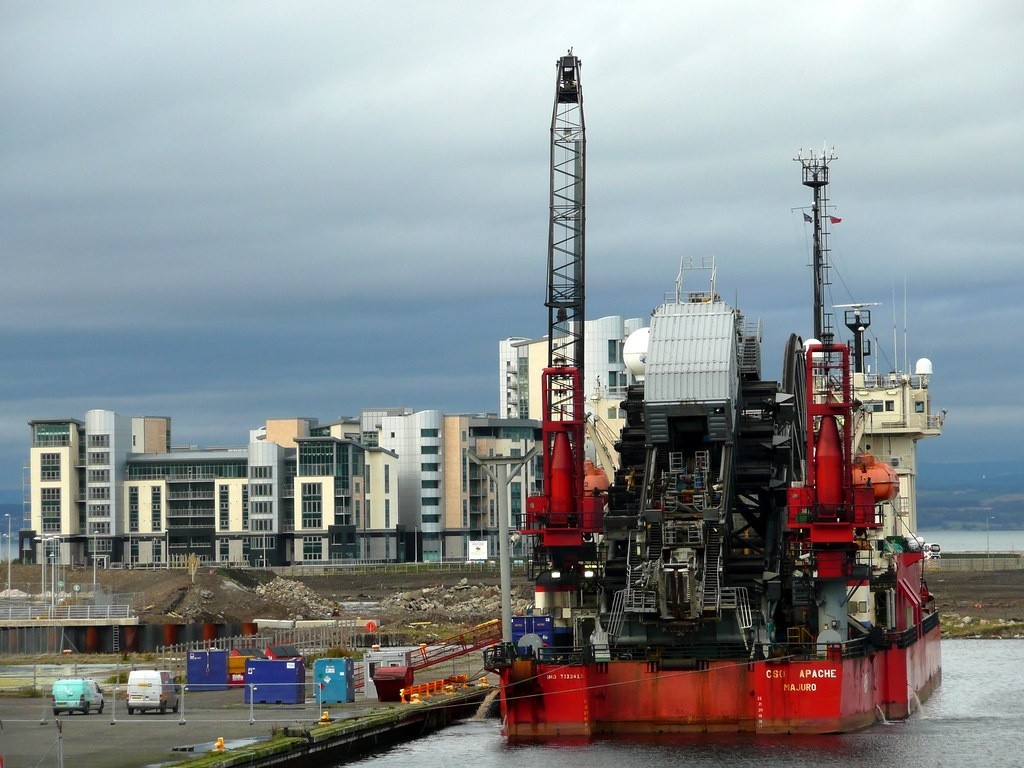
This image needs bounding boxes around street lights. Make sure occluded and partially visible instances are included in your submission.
[34,514,65,607]
[5,513,13,598]
[165,530,169,568]
[93,530,99,585]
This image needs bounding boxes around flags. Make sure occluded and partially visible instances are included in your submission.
[831,216,841,224]
[803,213,812,223]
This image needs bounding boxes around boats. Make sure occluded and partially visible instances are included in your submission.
[482,44,945,744]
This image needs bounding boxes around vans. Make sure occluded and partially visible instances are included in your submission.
[126,668,179,714]
[52,677,104,715]
